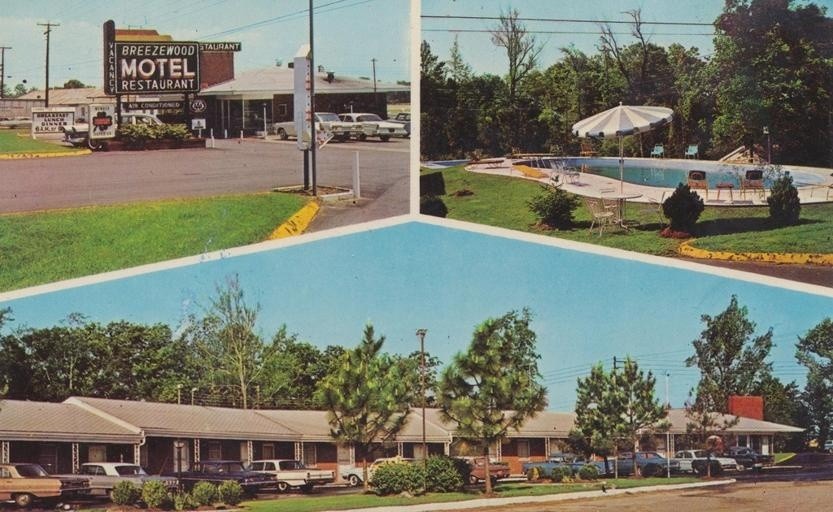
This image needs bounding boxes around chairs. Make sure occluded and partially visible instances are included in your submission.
[740,169,765,201]
[650,143,664,159]
[684,143,699,159]
[640,191,666,229]
[583,196,615,238]
[505,143,602,185]
[464,149,504,169]
[688,170,708,203]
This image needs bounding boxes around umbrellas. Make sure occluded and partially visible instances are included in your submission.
[571,101,675,196]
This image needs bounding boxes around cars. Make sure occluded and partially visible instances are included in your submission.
[451,446,774,484]
[801,438,833,453]
[274,112,410,142]
[0,458,415,508]
[62,112,162,150]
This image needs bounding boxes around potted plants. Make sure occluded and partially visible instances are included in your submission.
[105,119,206,151]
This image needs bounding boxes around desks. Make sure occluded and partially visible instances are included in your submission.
[602,193,642,231]
[715,182,733,201]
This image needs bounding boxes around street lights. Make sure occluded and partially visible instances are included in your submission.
[0,47,12,98]
[177,384,183,404]
[174,440,185,495]
[371,58,377,104]
[565,89,573,152]
[192,388,198,405]
[763,126,771,164]
[416,329,427,459]
